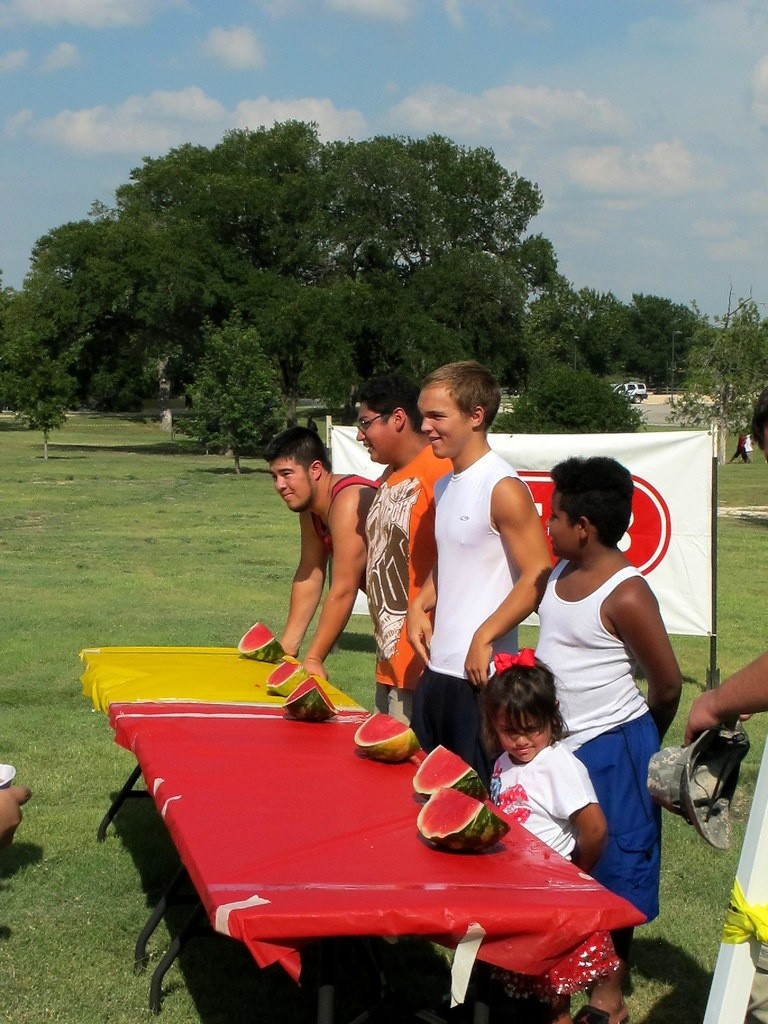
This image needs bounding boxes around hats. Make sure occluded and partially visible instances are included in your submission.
[647,721,750,850]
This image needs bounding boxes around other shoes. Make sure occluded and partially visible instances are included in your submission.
[572,1005,631,1024]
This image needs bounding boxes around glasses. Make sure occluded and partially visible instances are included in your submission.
[354,414,391,429]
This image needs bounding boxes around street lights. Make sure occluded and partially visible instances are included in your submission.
[671,331,683,407]
[574,336,579,371]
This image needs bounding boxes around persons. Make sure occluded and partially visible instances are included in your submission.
[407,358,552,796]
[480,456,682,1024]
[264,426,379,683]
[684,652,767,751]
[353,371,454,726]
[1,786,32,849]
[728,430,753,464]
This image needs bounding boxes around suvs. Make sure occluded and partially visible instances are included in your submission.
[612,383,648,404]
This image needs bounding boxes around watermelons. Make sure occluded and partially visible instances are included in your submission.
[417,787,511,852]
[237,622,288,661]
[412,744,489,803]
[353,712,421,763]
[282,677,339,722]
[266,661,309,697]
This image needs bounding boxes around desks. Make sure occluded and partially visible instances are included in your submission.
[80,647,647,1024]
